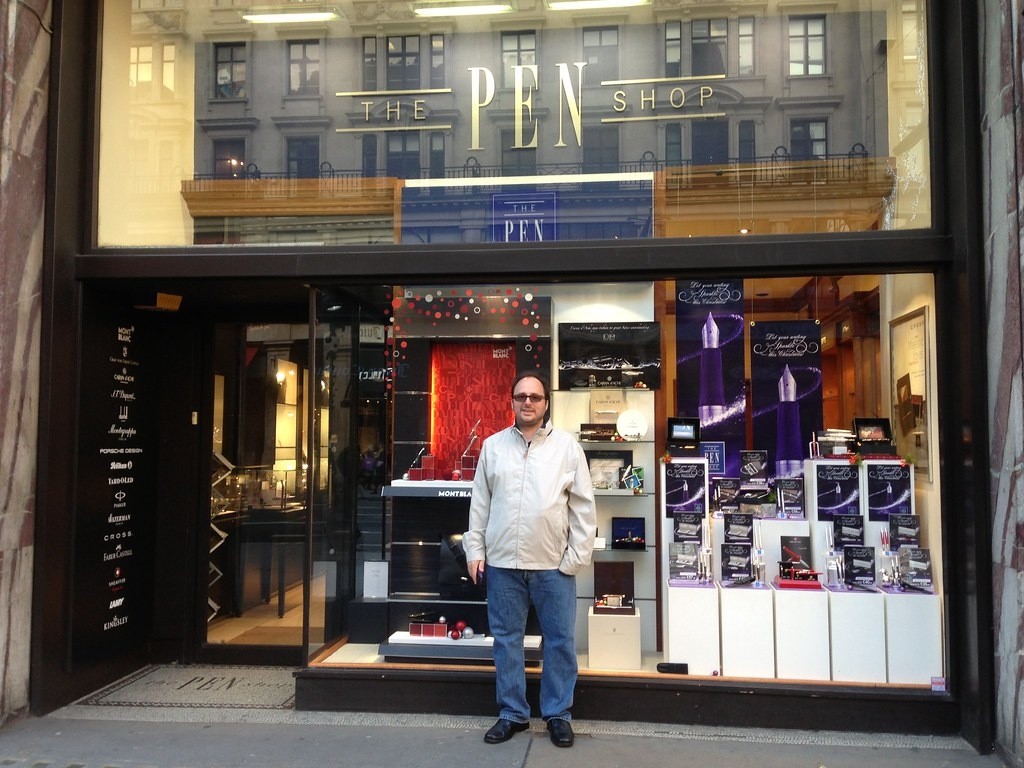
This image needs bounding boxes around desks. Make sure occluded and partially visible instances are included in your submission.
[588,457,944,687]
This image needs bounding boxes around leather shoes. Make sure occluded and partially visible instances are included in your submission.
[546,719,573,747]
[484,717,530,744]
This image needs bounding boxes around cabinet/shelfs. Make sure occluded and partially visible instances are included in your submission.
[571,388,653,553]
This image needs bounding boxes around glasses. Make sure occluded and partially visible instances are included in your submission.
[512,393,547,403]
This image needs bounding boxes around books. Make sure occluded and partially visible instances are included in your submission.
[780,536,811,576]
[740,450,769,490]
[898,548,934,594]
[721,544,751,581]
[774,478,804,520]
[867,463,912,522]
[844,544,877,590]
[724,514,753,547]
[665,463,706,519]
[888,513,921,552]
[674,511,702,550]
[817,465,860,522]
[834,513,864,556]
[669,543,707,584]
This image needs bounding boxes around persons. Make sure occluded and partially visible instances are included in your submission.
[462,371,597,746]
[712,477,740,513]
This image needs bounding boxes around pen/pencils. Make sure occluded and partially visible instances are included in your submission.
[683,481,688,503]
[698,310,726,423]
[887,483,893,505]
[783,546,809,568]
[460,436,478,459]
[411,448,425,468]
[715,485,721,510]
[776,365,803,477]
[470,420,481,437]
[835,483,842,506]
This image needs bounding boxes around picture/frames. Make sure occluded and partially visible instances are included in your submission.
[890,301,934,484]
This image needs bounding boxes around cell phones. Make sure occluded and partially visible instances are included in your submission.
[477,568,483,586]
